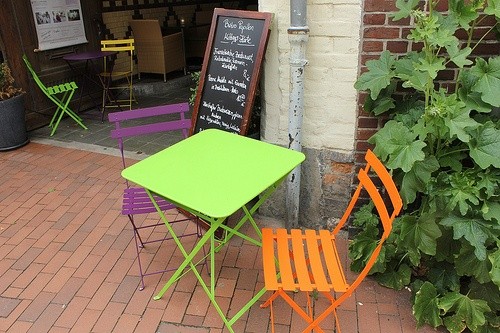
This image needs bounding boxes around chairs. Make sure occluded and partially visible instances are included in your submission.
[107,102,211,291]
[127,19,187,82]
[260,149,403,333]
[99,39,137,113]
[21,53,88,137]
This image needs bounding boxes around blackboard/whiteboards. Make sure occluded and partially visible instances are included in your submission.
[174,7,274,239]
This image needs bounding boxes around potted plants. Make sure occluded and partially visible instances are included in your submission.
[0,59,30,152]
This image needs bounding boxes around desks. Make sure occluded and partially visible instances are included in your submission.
[63,50,118,121]
[121,129,307,333]
